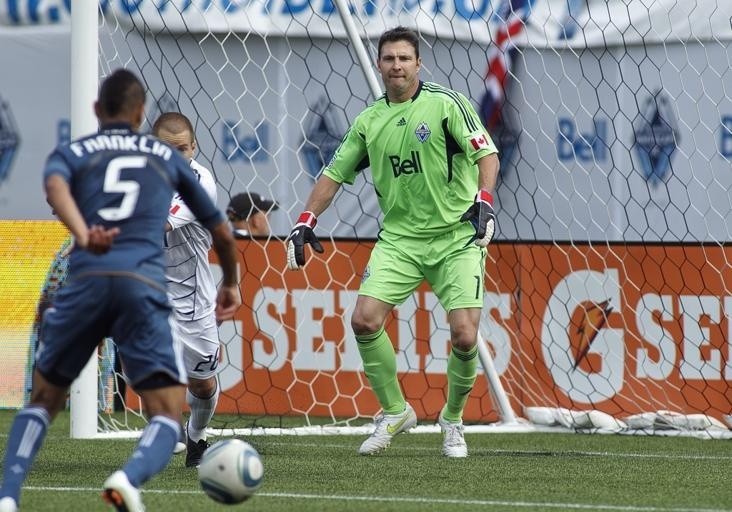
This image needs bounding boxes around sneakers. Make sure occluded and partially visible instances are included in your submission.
[185,420,210,468]
[438,409,468,460]
[102,469,145,512]
[360,402,417,455]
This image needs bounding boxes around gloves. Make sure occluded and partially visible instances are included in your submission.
[286,211,323,271]
[460,189,496,247]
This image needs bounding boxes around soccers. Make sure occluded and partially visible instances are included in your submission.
[197,438,265,504]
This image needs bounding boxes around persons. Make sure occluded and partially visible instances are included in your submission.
[226,192,278,237]
[1,68,241,512]
[287,28,500,458]
[154,114,216,467]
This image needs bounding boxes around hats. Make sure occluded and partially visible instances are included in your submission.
[226,193,279,222]
